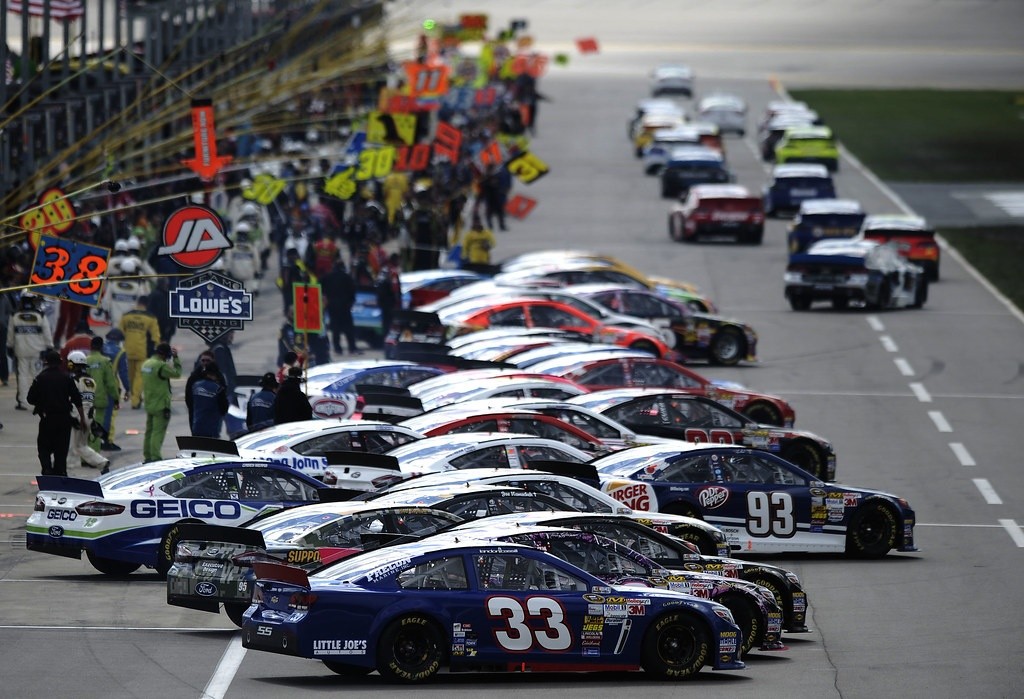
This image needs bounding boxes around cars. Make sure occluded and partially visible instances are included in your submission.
[627,60,942,284]
[22,241,923,682]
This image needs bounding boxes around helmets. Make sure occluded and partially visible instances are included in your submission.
[235,98,355,233]
[114,238,128,251]
[121,259,135,273]
[19,288,38,297]
[126,236,141,250]
[67,349,87,364]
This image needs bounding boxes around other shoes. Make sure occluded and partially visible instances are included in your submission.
[102,442,122,451]
[100,461,110,475]
[80,461,95,468]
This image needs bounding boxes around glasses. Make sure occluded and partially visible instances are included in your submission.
[199,359,211,362]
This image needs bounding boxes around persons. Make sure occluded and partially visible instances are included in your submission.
[27,350,88,476]
[67,350,110,475]
[141,343,182,463]
[0,35,546,451]
